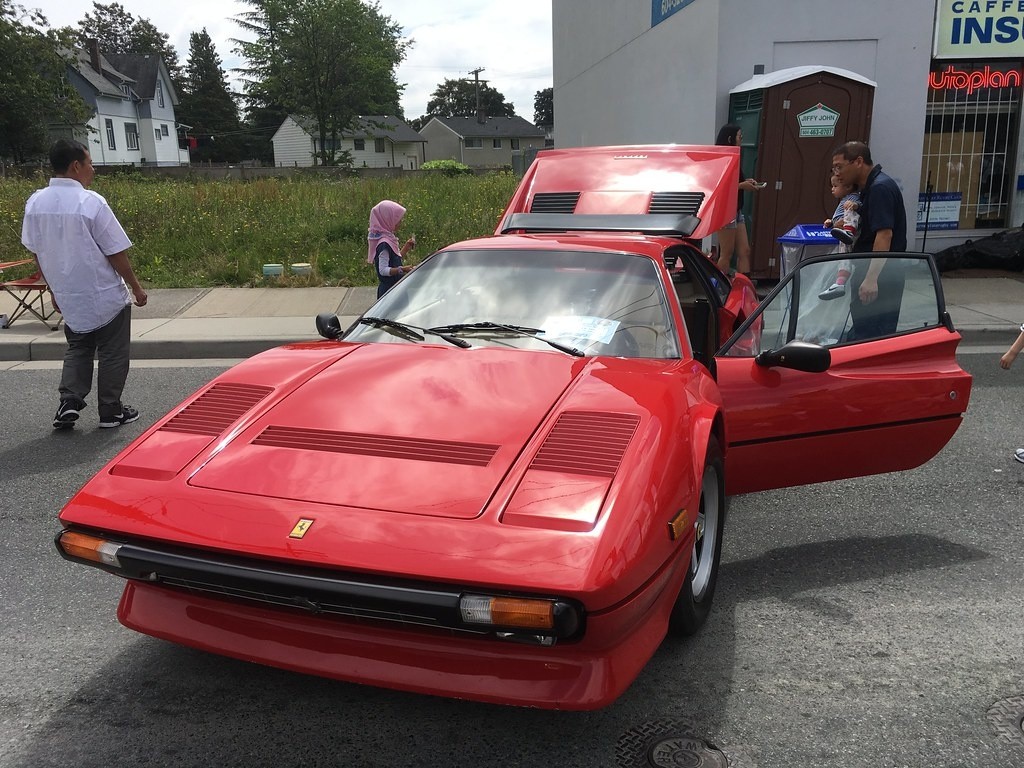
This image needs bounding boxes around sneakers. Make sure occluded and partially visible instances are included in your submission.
[98,404,140,427]
[830,228,854,245]
[53,399,80,429]
[818,284,845,300]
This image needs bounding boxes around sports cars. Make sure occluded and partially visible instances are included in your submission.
[53,144,974,713]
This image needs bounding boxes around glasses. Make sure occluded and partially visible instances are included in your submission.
[735,135,743,139]
[832,159,856,173]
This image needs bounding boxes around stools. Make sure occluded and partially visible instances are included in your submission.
[0,270,63,331]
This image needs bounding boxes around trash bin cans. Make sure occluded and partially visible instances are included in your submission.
[777,224,840,311]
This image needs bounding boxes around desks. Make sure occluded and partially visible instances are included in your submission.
[0,259,34,270]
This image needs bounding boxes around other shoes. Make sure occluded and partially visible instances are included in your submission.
[1014,448,1024,463]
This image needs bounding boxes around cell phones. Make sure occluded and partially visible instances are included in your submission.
[754,182,767,188]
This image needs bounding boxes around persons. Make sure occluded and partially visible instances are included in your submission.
[714,124,756,276]
[20,140,148,429]
[823,141,907,343]
[999,322,1024,464]
[367,199,417,319]
